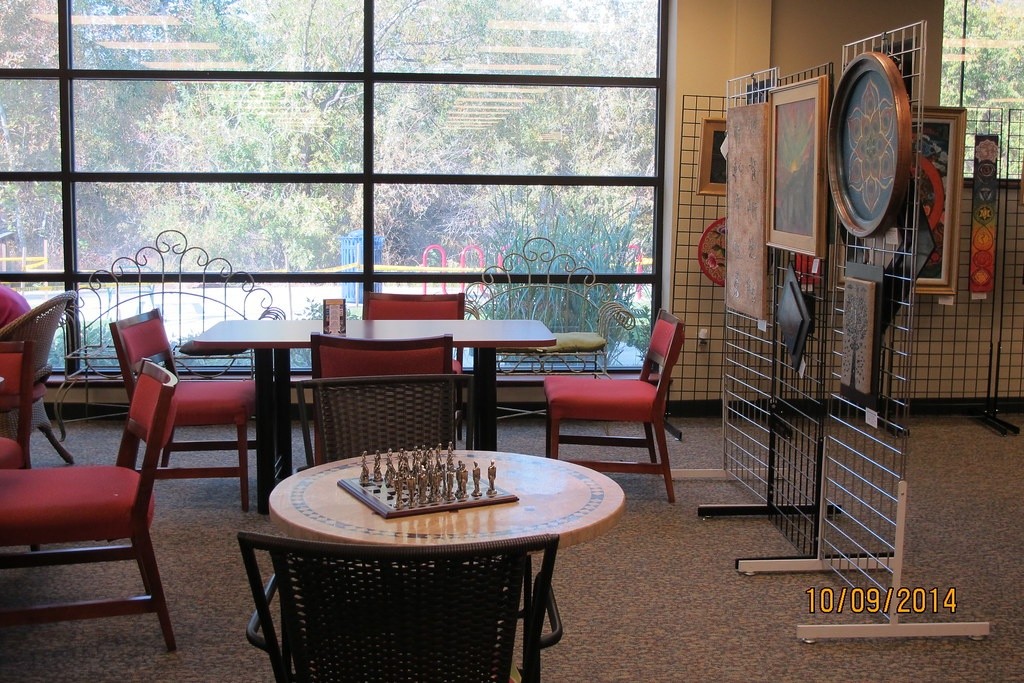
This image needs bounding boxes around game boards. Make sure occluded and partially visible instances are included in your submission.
[338,462,519,519]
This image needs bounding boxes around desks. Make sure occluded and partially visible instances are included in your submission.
[269,450,627,673]
[180,319,557,514]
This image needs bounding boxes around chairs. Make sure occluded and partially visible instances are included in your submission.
[361,291,465,440]
[289,330,474,473]
[544,308,684,503]
[237,530,564,683]
[0,339,43,552]
[108,308,254,512]
[0,289,77,465]
[463,237,636,435]
[0,358,179,652]
[53,229,287,442]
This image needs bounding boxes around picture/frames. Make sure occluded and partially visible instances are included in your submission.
[696,116,726,197]
[906,104,966,294]
[828,52,913,239]
[765,76,830,260]
